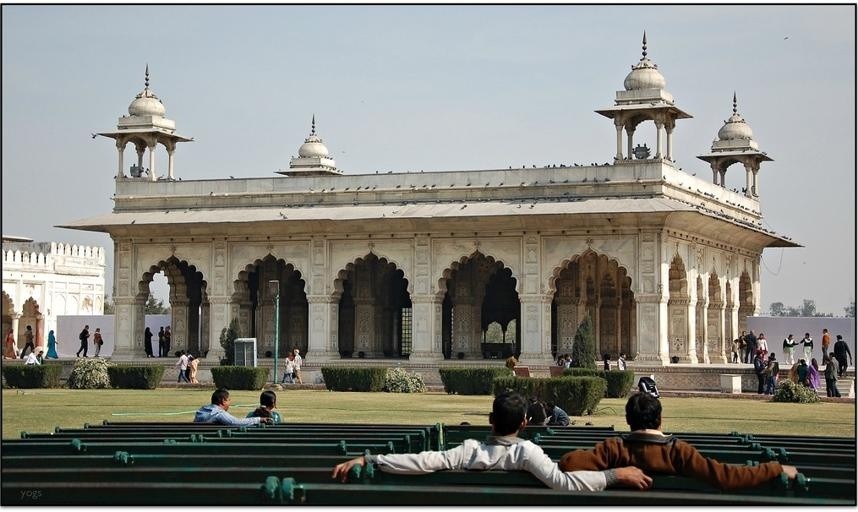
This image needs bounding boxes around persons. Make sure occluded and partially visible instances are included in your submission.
[557,390,798,490]
[506,352,518,377]
[546,400,571,426]
[245,390,282,423]
[192,388,274,426]
[524,395,547,426]
[563,353,573,368]
[555,355,565,366]
[617,351,628,370]
[281,350,296,383]
[292,348,303,385]
[330,390,656,491]
[728,329,854,397]
[603,353,612,371]
[4,323,201,384]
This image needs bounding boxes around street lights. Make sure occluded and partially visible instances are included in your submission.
[267,278,281,385]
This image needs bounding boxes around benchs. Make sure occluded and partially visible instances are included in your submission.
[1,420,856,507]
